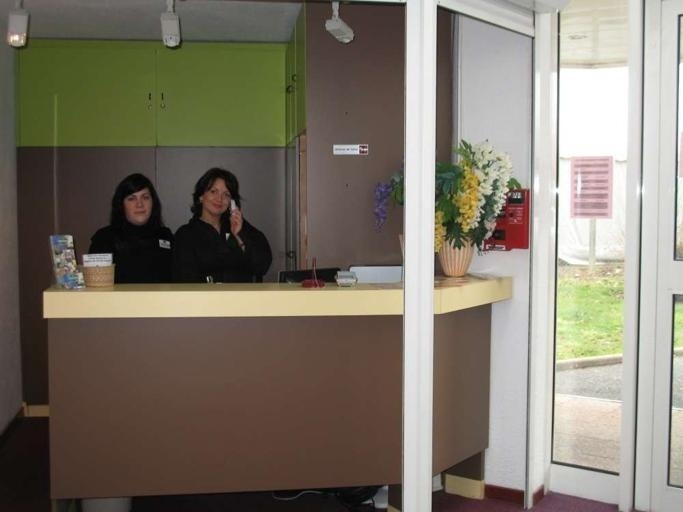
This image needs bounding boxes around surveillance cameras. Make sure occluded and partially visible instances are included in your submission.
[5,9,30,49]
[324,16,356,45]
[159,12,182,48]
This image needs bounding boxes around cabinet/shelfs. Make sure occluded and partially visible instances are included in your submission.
[286,2,308,148]
[15,39,285,148]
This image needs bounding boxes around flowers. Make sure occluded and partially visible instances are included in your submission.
[373,138,514,252]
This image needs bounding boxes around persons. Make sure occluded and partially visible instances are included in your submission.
[88,173,174,283]
[174,166,272,283]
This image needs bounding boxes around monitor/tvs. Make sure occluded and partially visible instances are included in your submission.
[348,263,402,285]
[279,267,341,286]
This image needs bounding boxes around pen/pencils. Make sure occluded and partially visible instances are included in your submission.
[312,258,316,279]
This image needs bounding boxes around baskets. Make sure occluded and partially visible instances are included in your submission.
[77,265,116,287]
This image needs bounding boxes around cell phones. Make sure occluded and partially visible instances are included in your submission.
[230,198,237,216]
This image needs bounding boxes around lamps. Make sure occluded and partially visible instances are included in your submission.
[8,0,31,49]
[325,2,355,44]
[161,0,182,48]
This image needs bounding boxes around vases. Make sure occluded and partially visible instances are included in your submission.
[437,230,474,278]
